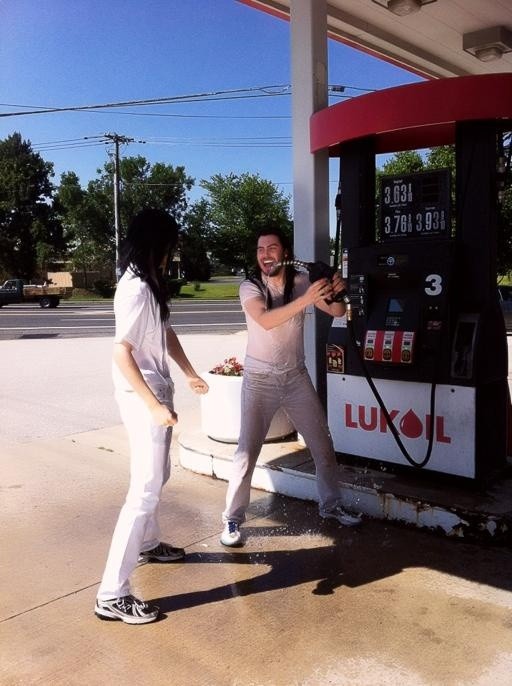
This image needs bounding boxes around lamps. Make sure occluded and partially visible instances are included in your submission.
[372,0,438,16]
[462,25,512,63]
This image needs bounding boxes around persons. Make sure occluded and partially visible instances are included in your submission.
[93,208,210,625]
[221,225,359,547]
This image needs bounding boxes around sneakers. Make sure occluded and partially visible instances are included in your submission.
[319,505,362,526]
[139,542,185,561]
[95,594,159,624]
[220,522,241,546]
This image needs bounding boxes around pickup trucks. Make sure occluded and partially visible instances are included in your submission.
[0,278,74,309]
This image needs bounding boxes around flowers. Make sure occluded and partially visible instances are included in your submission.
[208,355,245,376]
